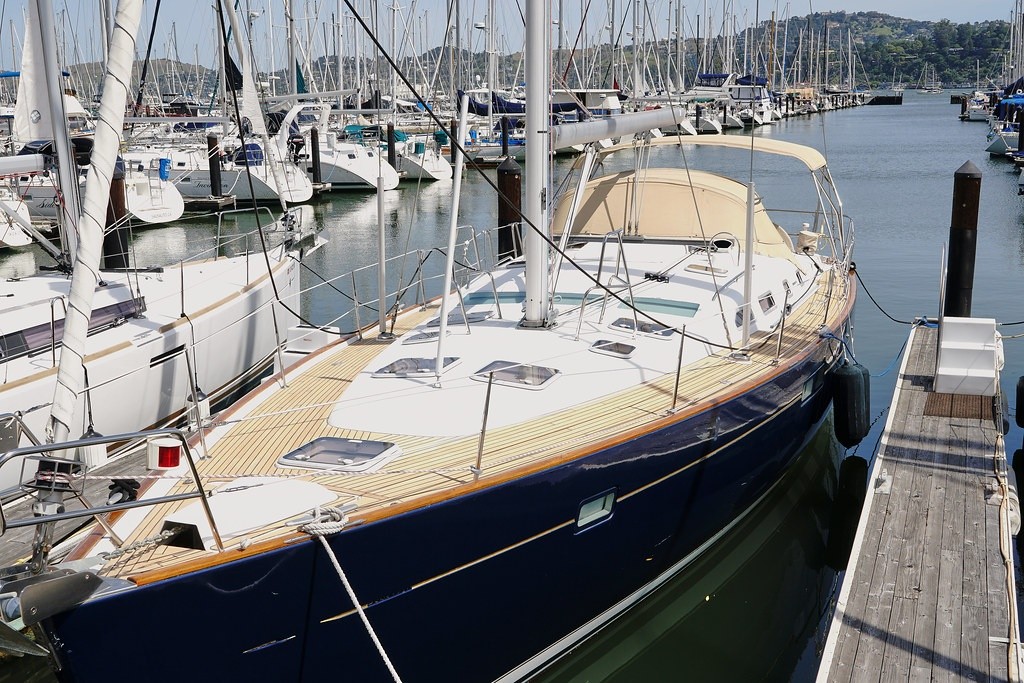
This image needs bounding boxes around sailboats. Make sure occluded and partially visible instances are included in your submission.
[0,0,1024,683]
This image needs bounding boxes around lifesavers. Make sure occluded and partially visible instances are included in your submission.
[1016,89,1022,94]
